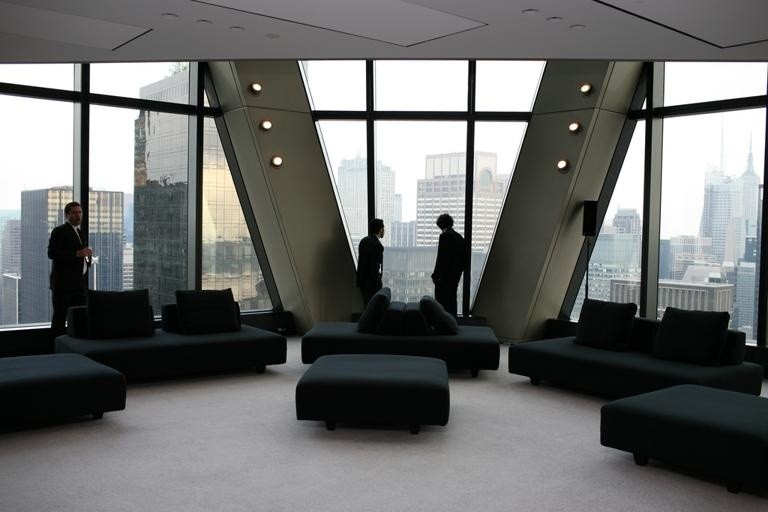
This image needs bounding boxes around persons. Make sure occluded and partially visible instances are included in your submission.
[357,218,385,309]
[46,201,92,355]
[430,214,468,316]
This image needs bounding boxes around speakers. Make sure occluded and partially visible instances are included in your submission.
[583,199,598,237]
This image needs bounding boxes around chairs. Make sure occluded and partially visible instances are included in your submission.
[405,301,500,377]
[301,301,405,364]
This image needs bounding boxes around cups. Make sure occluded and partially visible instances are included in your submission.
[85,248,93,263]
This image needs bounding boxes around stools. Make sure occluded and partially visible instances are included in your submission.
[296,355,450,435]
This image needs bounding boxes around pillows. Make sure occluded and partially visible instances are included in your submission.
[421,295,458,335]
[175,288,239,333]
[657,307,730,369]
[358,287,391,334]
[87,288,152,340]
[576,298,637,352]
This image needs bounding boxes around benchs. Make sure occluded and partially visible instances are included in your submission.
[600,384,768,500]
[0,354,127,430]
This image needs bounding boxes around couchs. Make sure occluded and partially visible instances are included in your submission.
[508,298,764,399]
[53,301,286,382]
